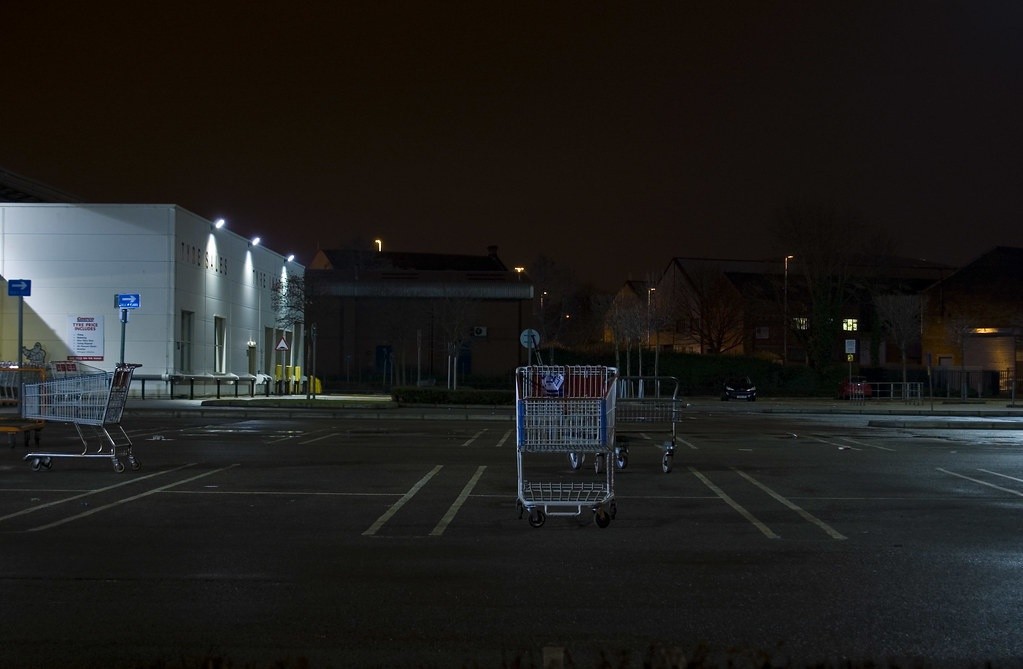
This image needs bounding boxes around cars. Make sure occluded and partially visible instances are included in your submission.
[720,374,767,395]
[839,375,873,402]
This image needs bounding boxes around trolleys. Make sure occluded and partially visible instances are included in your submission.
[569,376,682,474]
[19,362,143,474]
[514,364,620,529]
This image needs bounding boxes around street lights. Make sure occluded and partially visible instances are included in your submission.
[514,266,525,366]
[645,286,658,352]
[785,255,794,356]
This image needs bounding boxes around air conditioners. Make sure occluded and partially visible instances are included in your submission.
[473,326,486,337]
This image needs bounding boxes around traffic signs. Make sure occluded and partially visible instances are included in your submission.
[8,279,31,297]
[117,292,142,309]
